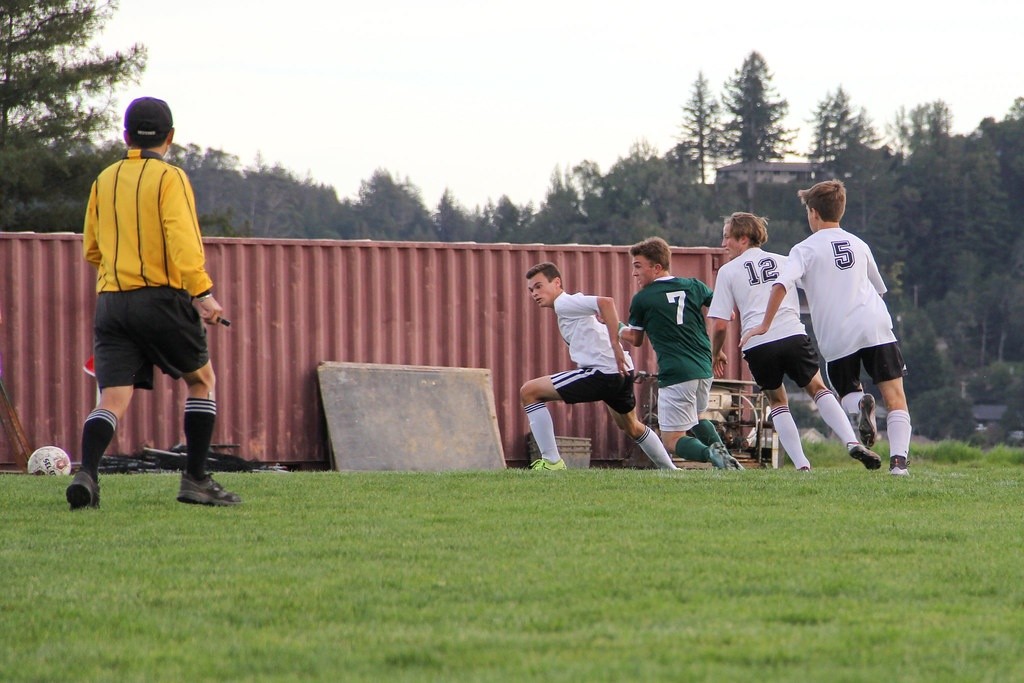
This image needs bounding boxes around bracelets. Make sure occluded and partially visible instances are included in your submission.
[197,293,213,302]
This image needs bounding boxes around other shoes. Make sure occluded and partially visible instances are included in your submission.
[709,442,745,471]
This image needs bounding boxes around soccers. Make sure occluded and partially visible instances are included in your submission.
[27,446,72,476]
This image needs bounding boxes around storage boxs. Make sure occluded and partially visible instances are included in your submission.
[527,434,592,469]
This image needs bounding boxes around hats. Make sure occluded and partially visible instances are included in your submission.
[124,98,172,140]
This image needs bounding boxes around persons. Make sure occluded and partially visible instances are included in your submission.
[66,97,242,506]
[706,211,881,470]
[618,237,746,470]
[737,179,912,475]
[519,261,680,470]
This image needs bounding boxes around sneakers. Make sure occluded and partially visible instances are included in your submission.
[530,458,567,471]
[889,456,910,475]
[177,471,242,506]
[847,441,881,469]
[797,467,810,473]
[67,471,100,511]
[858,394,877,446]
